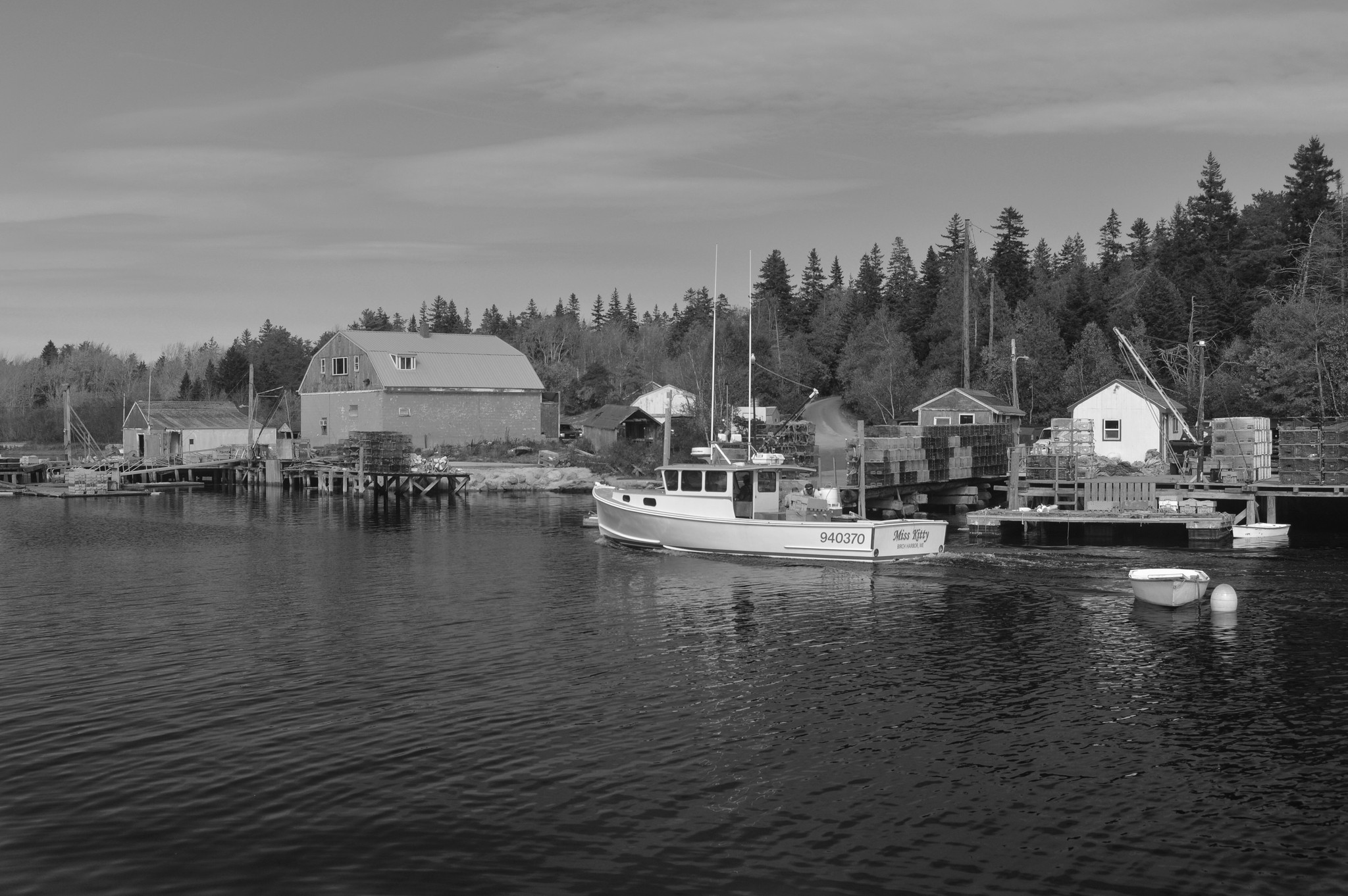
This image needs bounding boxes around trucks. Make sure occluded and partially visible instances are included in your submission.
[1034,426,1068,455]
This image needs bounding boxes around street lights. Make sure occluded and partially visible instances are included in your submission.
[725,381,729,443]
[1011,354,1030,445]
[239,404,253,484]
[663,389,693,466]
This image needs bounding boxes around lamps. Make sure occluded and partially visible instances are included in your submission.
[362,378,370,385]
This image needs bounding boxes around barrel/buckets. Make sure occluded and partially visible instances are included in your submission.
[819,487,838,506]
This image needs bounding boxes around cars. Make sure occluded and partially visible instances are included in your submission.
[559,424,582,443]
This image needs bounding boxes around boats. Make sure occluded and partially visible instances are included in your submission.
[1128,568,1210,606]
[592,244,949,564]
[1231,522,1291,538]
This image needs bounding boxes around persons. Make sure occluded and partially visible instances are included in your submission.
[801,483,822,500]
[784,487,803,509]
[738,475,753,502]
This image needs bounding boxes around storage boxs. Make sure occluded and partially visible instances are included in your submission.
[187,444,247,464]
[19,456,39,466]
[52,468,117,494]
[790,495,828,511]
[1087,496,1216,514]
[537,450,559,467]
[1221,470,1248,484]
